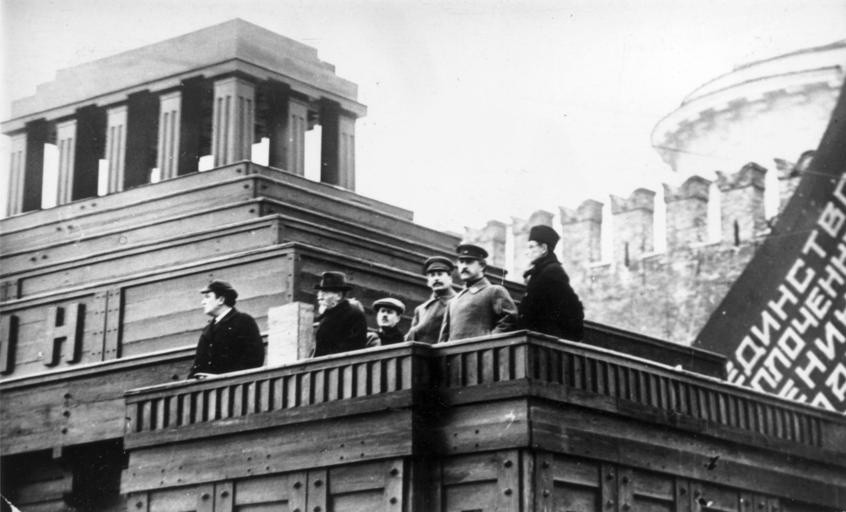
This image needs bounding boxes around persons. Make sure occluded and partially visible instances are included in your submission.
[346,298,382,347]
[514,223,569,333]
[436,243,519,344]
[367,297,406,346]
[186,280,266,381]
[402,254,458,345]
[307,270,367,359]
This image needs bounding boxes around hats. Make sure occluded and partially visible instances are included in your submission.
[456,244,488,260]
[422,256,454,275]
[200,281,235,294]
[372,298,406,315]
[529,225,560,246]
[314,271,354,291]
